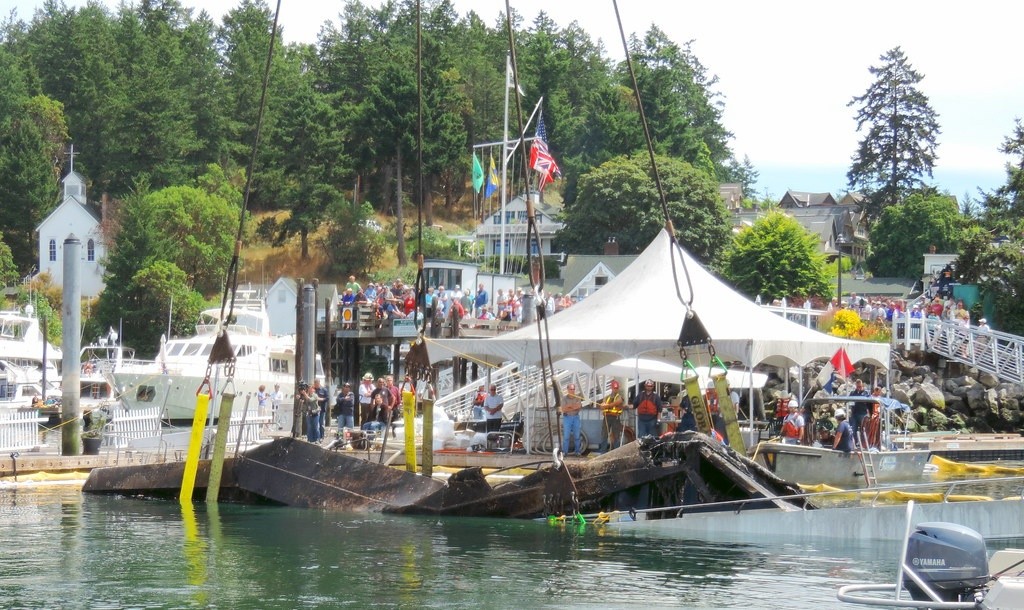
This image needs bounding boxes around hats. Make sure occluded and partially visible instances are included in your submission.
[363,373,374,381]
[979,318,986,323]
[913,305,918,308]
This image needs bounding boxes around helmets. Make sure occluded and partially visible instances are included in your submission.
[834,409,845,416]
[612,381,619,388]
[788,400,798,408]
[645,379,654,385]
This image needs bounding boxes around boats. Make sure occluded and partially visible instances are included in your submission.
[0,47,617,465]
[741,393,932,489]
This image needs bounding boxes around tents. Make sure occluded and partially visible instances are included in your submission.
[559,354,768,392]
[422,224,889,449]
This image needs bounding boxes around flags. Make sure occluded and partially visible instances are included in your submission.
[485,156,500,200]
[528,100,562,190]
[472,152,484,193]
[831,349,855,377]
[816,360,835,394]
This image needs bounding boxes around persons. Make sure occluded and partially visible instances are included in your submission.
[484,384,504,434]
[296,378,355,444]
[359,372,401,446]
[845,378,868,444]
[832,409,853,453]
[560,383,584,456]
[599,380,624,454]
[258,385,273,433]
[32,393,39,406]
[628,378,662,437]
[778,400,805,445]
[473,385,484,420]
[716,380,740,445]
[337,274,572,330]
[828,292,990,360]
[269,383,283,423]
[817,426,835,449]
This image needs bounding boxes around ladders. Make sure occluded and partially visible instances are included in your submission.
[857,431,878,487]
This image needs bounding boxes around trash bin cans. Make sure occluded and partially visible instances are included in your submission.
[522,295,535,326]
[903,523,989,610]
[988,547,1024,578]
[81,434,103,455]
[976,576,1024,610]
